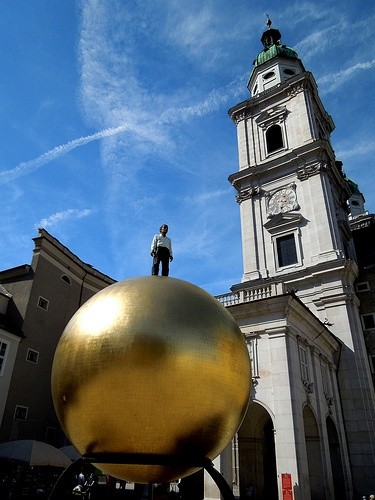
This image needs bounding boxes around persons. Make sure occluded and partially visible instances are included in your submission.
[151,224,173,276]
